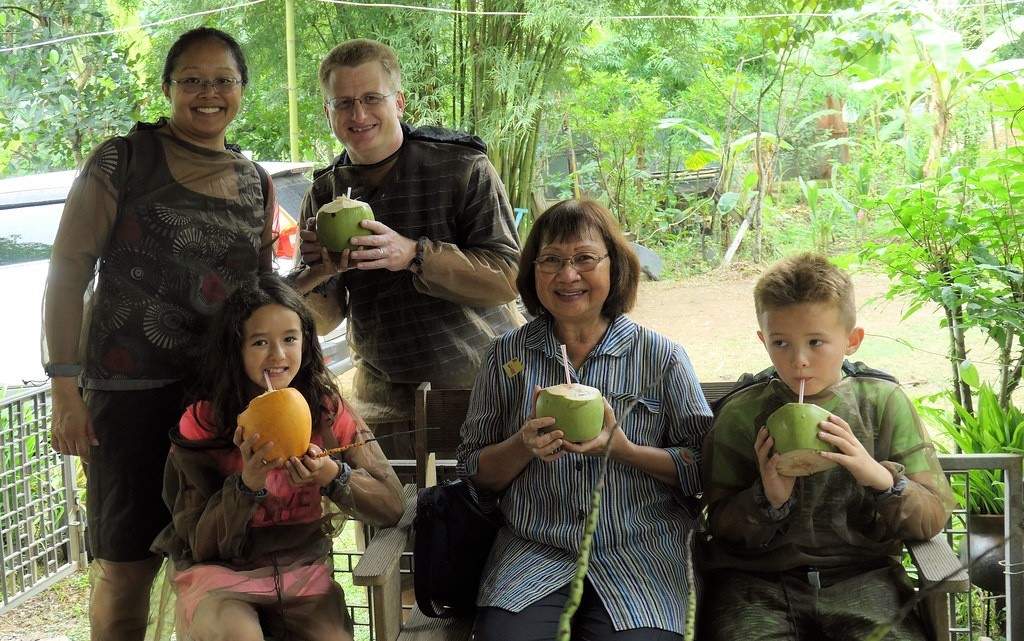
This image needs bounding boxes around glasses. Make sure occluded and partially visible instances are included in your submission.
[325,91,398,113]
[169,77,243,93]
[532,251,610,274]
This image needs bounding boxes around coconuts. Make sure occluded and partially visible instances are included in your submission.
[766,403,841,476]
[238,388,313,469]
[315,194,375,253]
[536,382,605,443]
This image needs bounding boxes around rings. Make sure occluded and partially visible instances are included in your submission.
[334,264,344,274]
[377,247,384,259]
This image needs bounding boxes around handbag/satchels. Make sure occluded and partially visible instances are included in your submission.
[413,479,501,619]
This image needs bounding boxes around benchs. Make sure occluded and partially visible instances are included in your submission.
[352,382,969,641]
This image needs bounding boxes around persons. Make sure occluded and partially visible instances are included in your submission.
[454,198,716,641]
[699,251,958,641]
[39,26,368,641]
[292,38,530,641]
[147,272,407,641]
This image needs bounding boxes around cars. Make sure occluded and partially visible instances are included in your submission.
[0,162,352,392]
